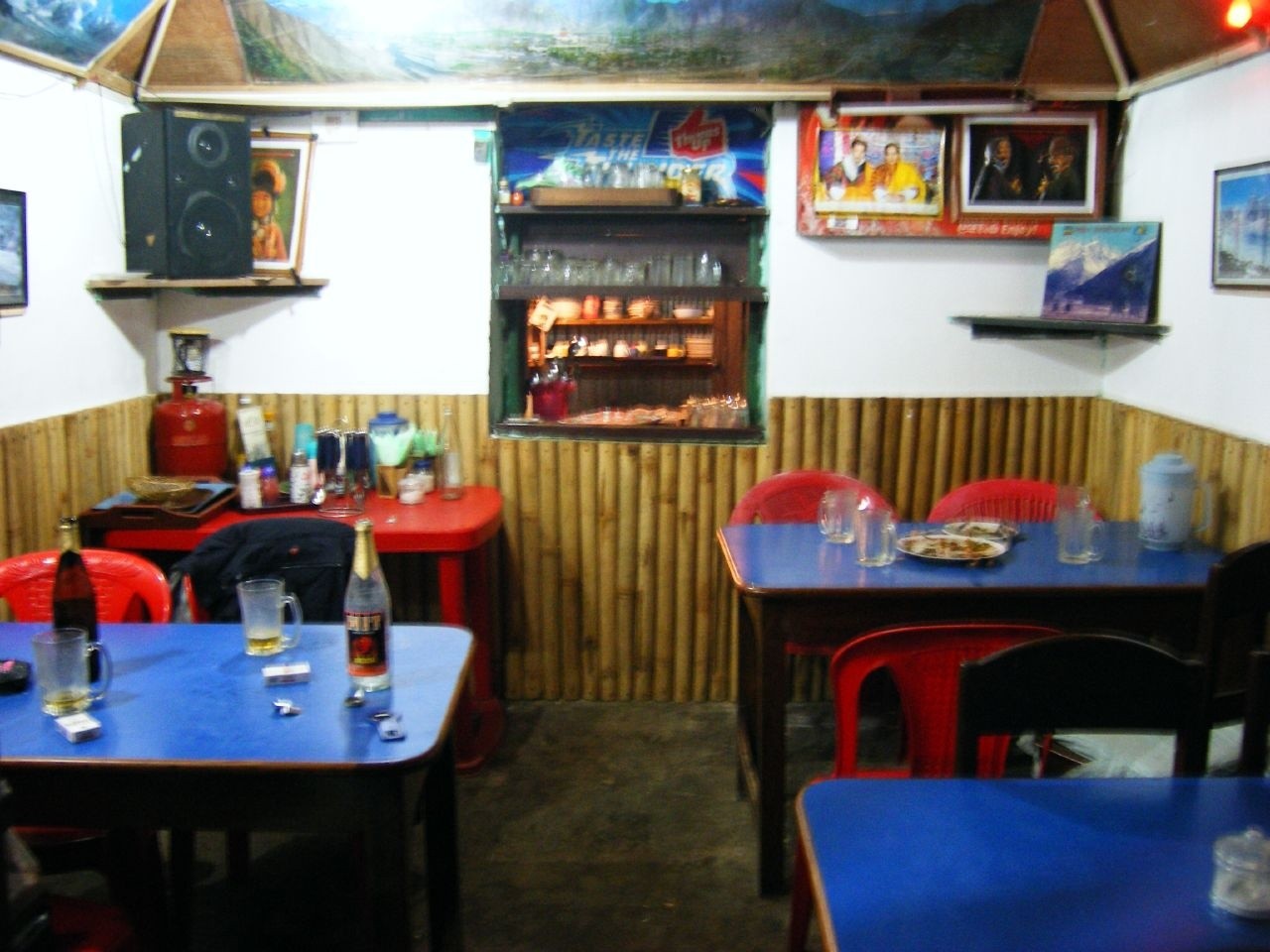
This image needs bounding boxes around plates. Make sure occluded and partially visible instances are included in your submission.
[894,521,1020,564]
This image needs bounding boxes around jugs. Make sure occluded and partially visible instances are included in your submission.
[1136,455,1216,552]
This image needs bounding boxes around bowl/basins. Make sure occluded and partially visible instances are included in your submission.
[671,308,703,319]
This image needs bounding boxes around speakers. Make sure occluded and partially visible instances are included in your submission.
[121,109,254,282]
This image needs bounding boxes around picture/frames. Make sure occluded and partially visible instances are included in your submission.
[952,112,1108,222]
[1211,160,1269,290]
[250,130,316,276]
[1,186,29,307]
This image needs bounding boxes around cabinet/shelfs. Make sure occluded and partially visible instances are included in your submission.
[494,209,765,440]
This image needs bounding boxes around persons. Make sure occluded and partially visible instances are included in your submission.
[825,137,926,204]
[970,138,1084,204]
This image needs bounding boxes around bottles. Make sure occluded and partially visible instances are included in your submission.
[499,179,525,206]
[680,166,703,204]
[342,520,393,694]
[50,514,104,682]
[397,458,435,505]
[1209,829,1270,921]
[290,450,309,504]
[237,393,285,509]
[367,413,408,488]
[439,409,459,490]
[583,293,624,320]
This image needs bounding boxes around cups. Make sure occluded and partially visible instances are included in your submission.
[1052,486,1108,566]
[499,246,727,286]
[857,504,899,568]
[561,160,663,189]
[443,454,461,501]
[31,627,114,718]
[237,577,305,654]
[814,484,859,544]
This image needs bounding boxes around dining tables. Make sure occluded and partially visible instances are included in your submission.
[717,522,1227,896]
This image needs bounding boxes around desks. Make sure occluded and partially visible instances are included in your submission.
[1,622,479,952]
[106,482,504,767]
[791,780,1270,952]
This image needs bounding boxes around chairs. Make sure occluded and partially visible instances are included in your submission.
[808,626,1072,783]
[728,469,904,657]
[955,632,1212,779]
[926,478,1105,524]
[0,548,214,952]
[1201,535,1270,779]
